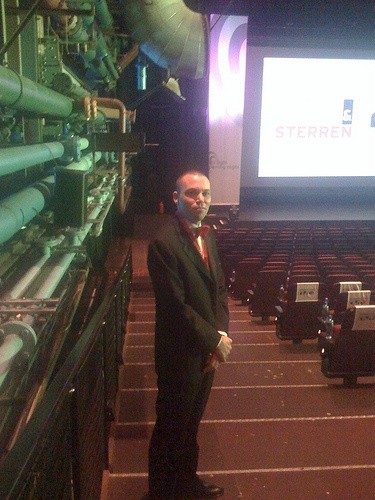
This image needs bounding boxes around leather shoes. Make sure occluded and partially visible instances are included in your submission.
[192,480,226,498]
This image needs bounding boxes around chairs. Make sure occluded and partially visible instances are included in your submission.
[212,225,375,387]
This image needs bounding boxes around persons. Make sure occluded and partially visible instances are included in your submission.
[147,170,232,500]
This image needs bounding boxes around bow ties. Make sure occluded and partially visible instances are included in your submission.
[189,225,210,240]
